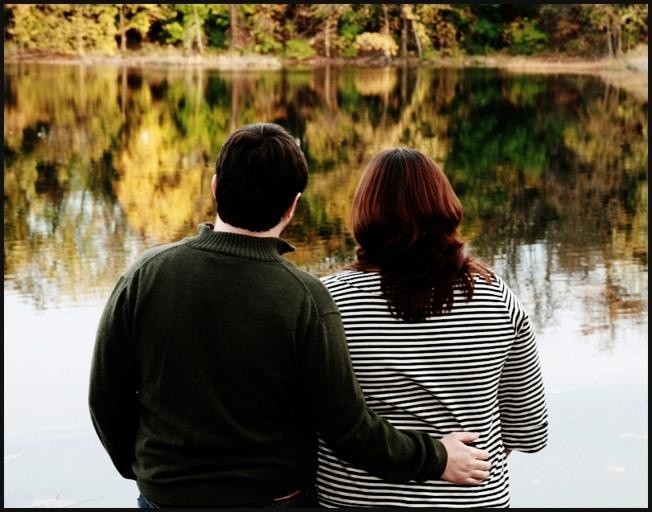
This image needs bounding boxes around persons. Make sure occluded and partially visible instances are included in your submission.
[315,147,547,509]
[89,123,491,511]
